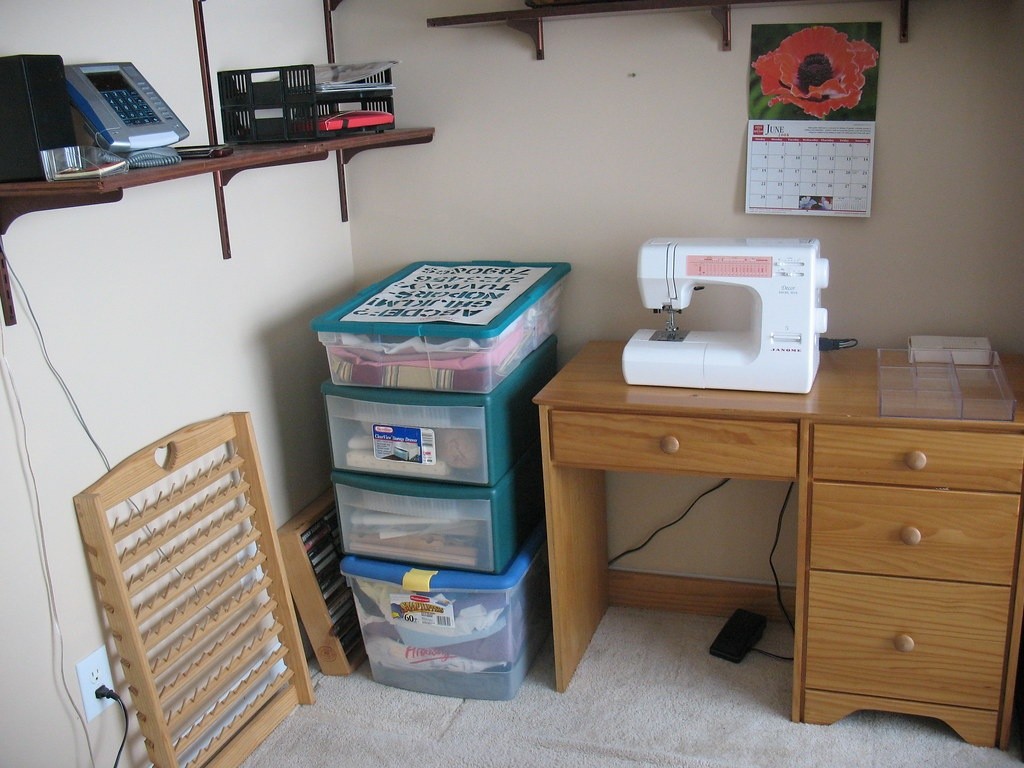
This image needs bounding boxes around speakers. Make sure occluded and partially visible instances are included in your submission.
[0,54,80,183]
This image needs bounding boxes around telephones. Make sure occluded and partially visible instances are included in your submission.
[64,63,190,168]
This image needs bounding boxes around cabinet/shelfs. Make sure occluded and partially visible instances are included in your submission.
[530,340,1024,753]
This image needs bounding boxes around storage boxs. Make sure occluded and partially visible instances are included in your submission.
[874,346,1018,426]
[216,63,397,143]
[329,440,544,574]
[319,335,559,485]
[906,333,993,367]
[309,260,572,396]
[340,518,548,702]
